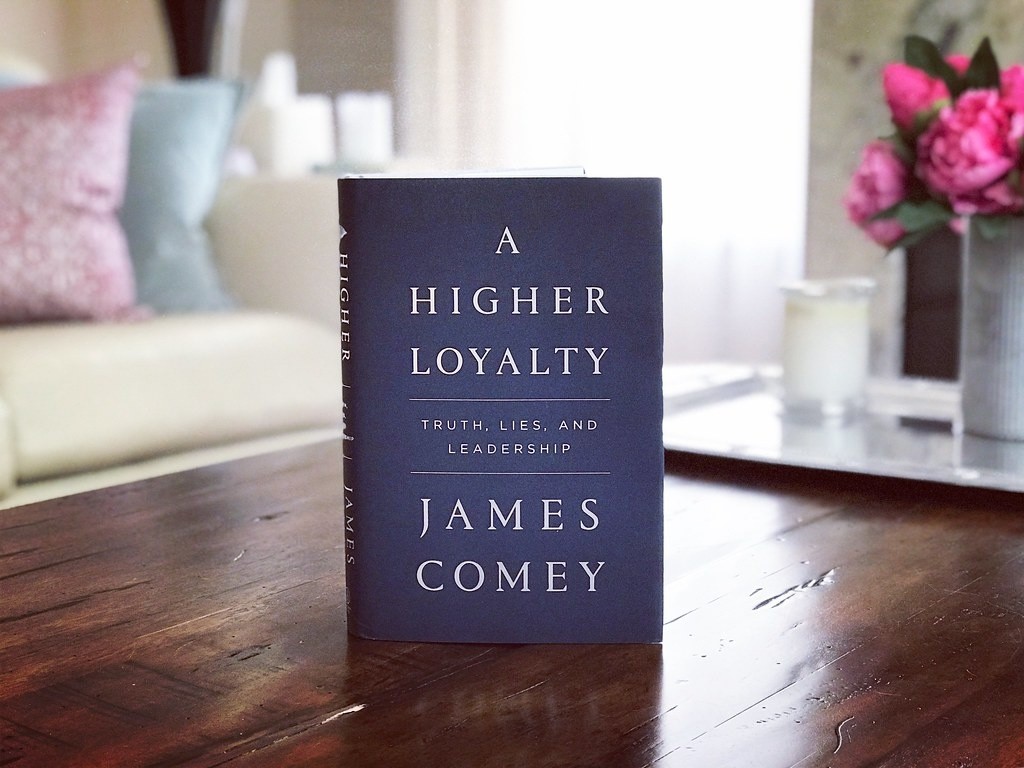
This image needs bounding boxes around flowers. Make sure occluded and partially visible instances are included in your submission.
[844,31,1024,256]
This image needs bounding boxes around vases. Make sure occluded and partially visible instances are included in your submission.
[954,209,1024,482]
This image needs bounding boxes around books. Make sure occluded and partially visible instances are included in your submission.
[337,177,664,643]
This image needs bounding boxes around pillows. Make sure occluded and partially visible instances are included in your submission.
[1,53,145,327]
[116,74,246,316]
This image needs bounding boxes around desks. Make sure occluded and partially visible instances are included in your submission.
[0,433,1024,768]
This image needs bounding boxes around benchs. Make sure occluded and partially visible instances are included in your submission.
[0,169,396,510]
